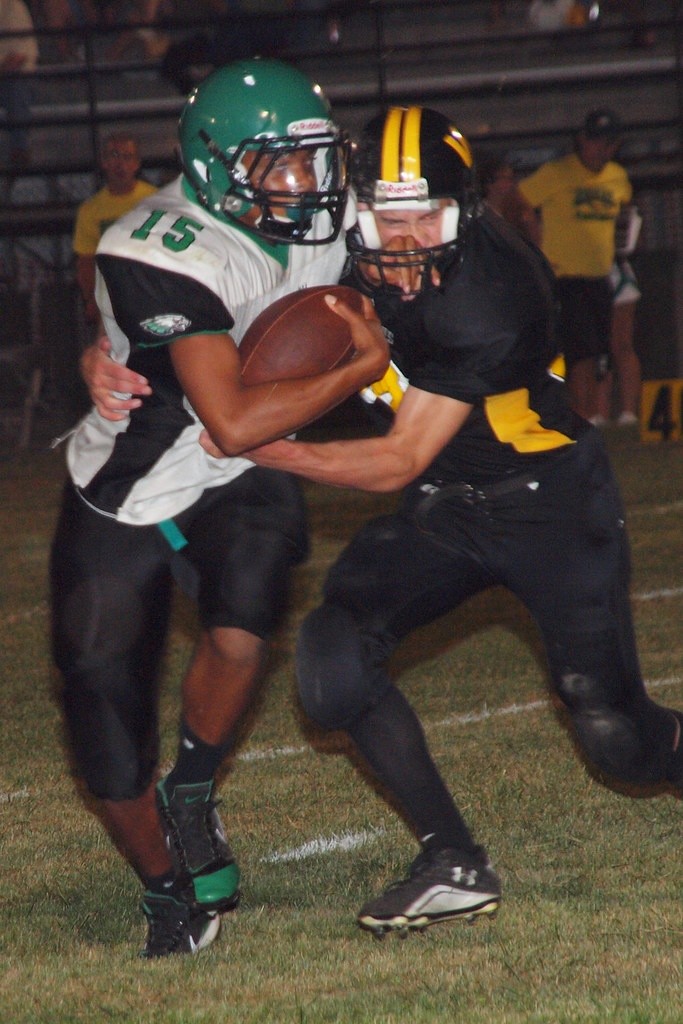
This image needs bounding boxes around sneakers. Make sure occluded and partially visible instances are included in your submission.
[138,890,222,959]
[356,842,503,938]
[156,772,241,912]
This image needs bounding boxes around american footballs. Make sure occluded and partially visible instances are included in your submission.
[238,284,364,387]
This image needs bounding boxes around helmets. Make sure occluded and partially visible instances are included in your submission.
[346,104,476,293]
[176,56,340,245]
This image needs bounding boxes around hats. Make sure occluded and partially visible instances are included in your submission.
[584,106,622,149]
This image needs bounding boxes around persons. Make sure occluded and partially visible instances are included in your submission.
[73,107,644,428]
[49,60,392,958]
[77,104,681,938]
[0,0,341,164]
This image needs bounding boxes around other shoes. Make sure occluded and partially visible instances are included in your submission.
[618,410,635,424]
[590,414,609,428]
